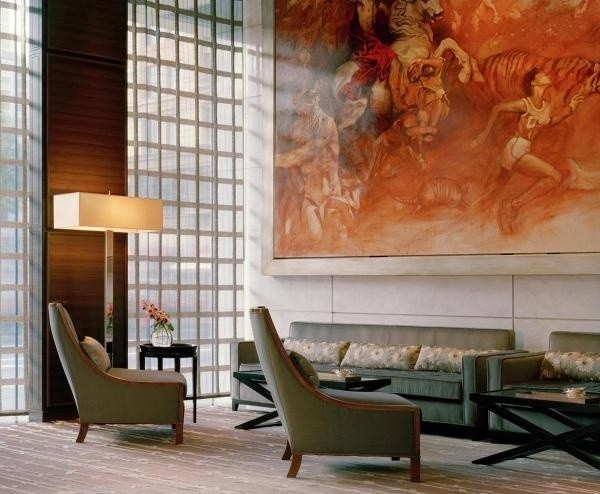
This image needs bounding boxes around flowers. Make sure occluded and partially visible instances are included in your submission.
[143,300,180,327]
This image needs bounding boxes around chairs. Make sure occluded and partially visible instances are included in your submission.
[46,300,191,446]
[251,306,421,483]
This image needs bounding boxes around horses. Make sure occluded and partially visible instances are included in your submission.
[317,0,484,133]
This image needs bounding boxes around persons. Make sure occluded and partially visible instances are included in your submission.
[474,61,586,235]
[358,0,410,112]
[277,83,349,249]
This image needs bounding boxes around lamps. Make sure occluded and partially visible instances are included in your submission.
[53,187,165,367]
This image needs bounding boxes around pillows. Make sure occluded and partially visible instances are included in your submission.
[539,347,600,384]
[342,339,418,374]
[286,348,325,390]
[415,340,498,378]
[81,327,114,375]
[275,330,351,368]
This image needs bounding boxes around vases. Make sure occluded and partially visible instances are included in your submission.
[153,321,175,347]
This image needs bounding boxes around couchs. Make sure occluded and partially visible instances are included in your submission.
[487,327,599,450]
[229,319,515,432]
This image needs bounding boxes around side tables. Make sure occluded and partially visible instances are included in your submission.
[139,340,197,423]
